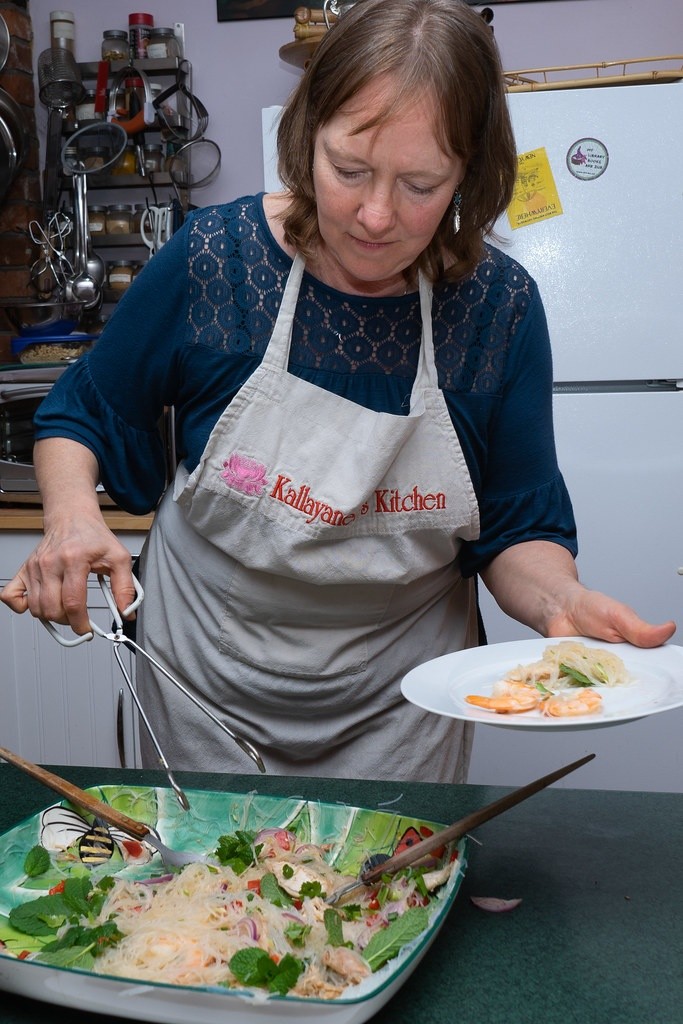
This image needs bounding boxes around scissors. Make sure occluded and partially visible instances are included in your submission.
[140,204,169,261]
[27,209,77,293]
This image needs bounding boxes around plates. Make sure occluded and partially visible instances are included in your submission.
[398,634,682,731]
[0,784,473,1024]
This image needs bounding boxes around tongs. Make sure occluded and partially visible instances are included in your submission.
[36,571,267,812]
[26,209,75,303]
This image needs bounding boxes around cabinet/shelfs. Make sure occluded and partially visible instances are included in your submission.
[43,57,193,303]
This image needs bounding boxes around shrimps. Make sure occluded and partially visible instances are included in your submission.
[463,679,603,720]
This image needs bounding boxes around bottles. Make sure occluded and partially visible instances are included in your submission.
[50,9,183,290]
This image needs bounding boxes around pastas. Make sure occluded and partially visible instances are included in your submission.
[93,832,416,999]
[511,640,634,690]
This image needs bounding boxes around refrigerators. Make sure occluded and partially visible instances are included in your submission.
[453,79,683,794]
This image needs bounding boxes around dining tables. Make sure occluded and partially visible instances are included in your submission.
[2,759,683,1024]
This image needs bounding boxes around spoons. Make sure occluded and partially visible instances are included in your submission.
[326,754,597,904]
[0,747,225,880]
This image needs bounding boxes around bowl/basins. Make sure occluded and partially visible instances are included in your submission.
[11,332,101,365]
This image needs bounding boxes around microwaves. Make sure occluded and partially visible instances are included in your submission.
[1,361,109,492]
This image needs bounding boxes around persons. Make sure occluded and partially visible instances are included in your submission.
[0,2,679,786]
